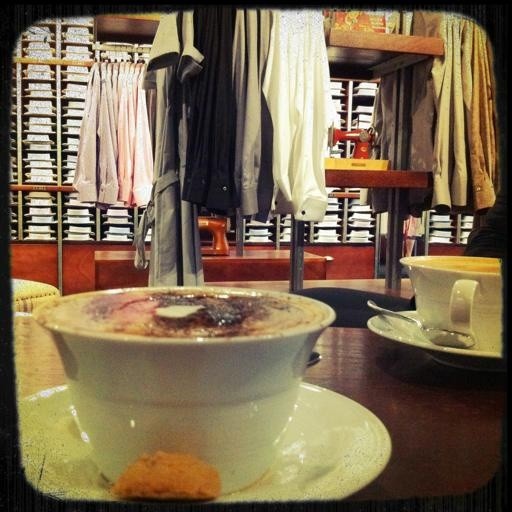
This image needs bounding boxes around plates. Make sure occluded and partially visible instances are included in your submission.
[17,380,392,501]
[368,310,504,375]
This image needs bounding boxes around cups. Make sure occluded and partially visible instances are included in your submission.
[390,9,414,35]
[401,256,503,355]
[30,287,337,499]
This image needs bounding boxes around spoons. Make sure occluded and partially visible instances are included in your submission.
[367,301,475,349]
